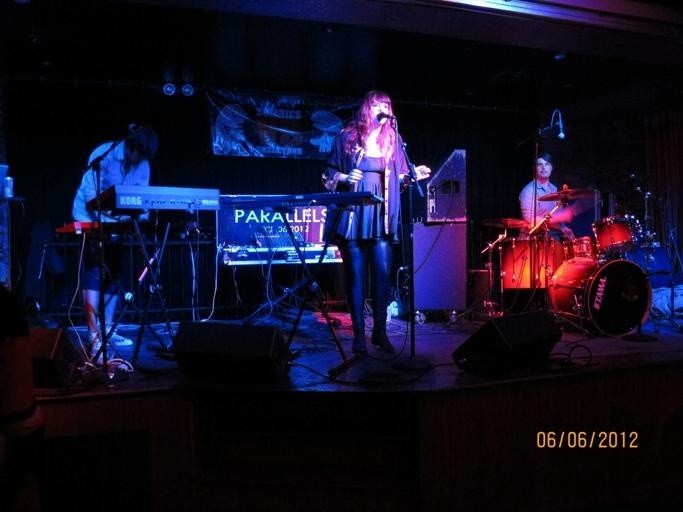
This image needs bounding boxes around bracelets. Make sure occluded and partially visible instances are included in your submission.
[0,398,38,426]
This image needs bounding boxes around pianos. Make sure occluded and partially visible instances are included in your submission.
[225,191,383,210]
[55,184,220,236]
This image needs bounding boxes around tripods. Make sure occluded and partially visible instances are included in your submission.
[389,118,433,370]
[641,249,683,331]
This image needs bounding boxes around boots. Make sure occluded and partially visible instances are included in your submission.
[369,318,396,354]
[350,322,368,355]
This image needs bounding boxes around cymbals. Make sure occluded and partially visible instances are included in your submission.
[538,186,596,199]
[481,217,528,229]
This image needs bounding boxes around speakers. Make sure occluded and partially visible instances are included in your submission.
[452,308,563,376]
[27,327,85,385]
[173,322,295,399]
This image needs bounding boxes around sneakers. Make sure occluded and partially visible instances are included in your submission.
[103,332,135,348]
[82,332,104,351]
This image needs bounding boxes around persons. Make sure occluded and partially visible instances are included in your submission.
[519,152,576,242]
[320,89,432,354]
[71,123,153,351]
[0,334,58,512]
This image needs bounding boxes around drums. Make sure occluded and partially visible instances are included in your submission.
[547,256,652,338]
[501,236,565,290]
[591,213,639,256]
[562,235,607,264]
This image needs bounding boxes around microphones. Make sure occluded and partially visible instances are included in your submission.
[377,112,397,121]
[128,123,146,136]
[557,112,566,139]
